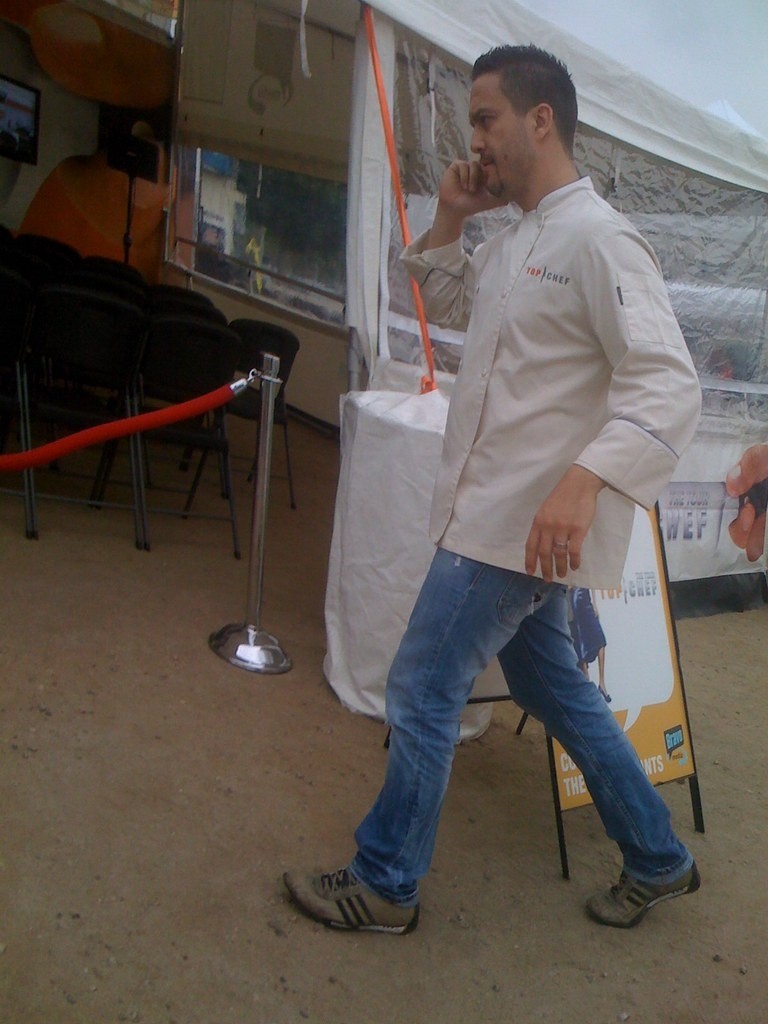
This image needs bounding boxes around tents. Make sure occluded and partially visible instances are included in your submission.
[345,2,767,584]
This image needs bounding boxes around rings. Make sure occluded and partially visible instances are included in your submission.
[553,542,568,549]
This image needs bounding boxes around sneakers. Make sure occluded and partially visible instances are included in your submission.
[585,860,702,929]
[282,865,418,935]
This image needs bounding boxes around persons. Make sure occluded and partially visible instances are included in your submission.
[284,42,703,934]
[567,585,611,702]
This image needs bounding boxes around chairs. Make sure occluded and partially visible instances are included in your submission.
[0,225,303,563]
